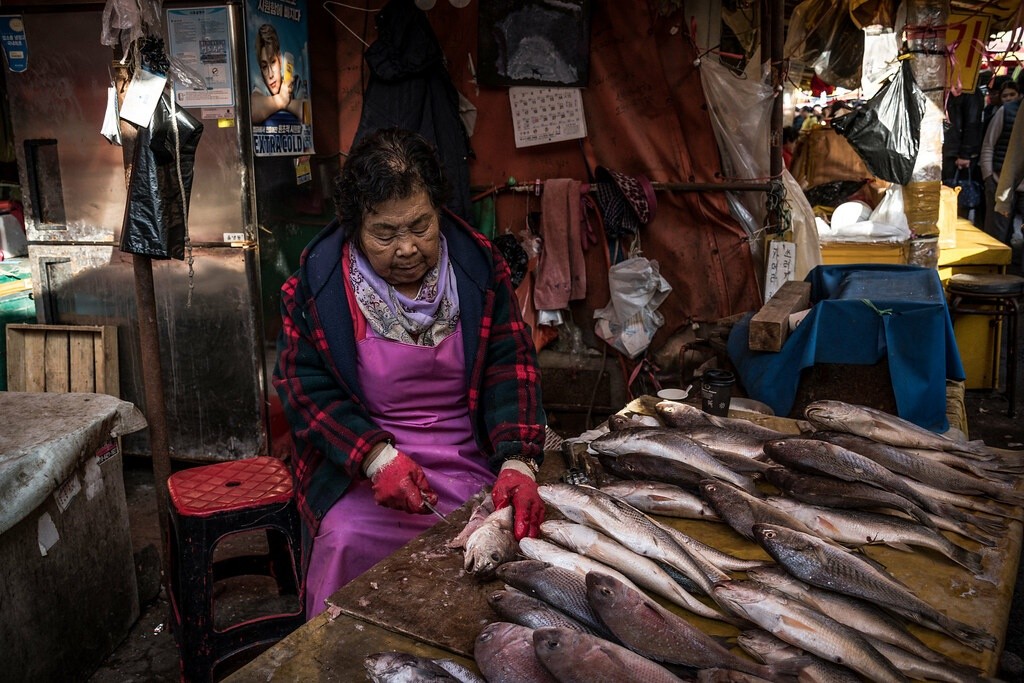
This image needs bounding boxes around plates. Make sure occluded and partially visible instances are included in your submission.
[657,388,689,400]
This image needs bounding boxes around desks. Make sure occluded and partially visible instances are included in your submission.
[221,394,1023,682]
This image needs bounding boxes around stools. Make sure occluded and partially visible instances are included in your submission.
[943,273,1024,451]
[167,458,309,683]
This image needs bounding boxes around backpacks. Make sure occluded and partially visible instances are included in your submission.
[827,58,928,188]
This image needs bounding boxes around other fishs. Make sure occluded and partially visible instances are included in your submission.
[362,397,1023,683]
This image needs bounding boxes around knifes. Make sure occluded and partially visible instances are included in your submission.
[422,495,457,528]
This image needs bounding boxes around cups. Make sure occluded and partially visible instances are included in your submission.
[701,368,735,418]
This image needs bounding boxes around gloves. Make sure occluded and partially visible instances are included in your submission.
[364,443,440,515]
[490,459,547,542]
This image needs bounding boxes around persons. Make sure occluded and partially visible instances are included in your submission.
[271,129,547,623]
[249,23,309,125]
[781,74,1024,276]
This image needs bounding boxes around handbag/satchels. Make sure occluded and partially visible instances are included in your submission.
[942,167,984,210]
